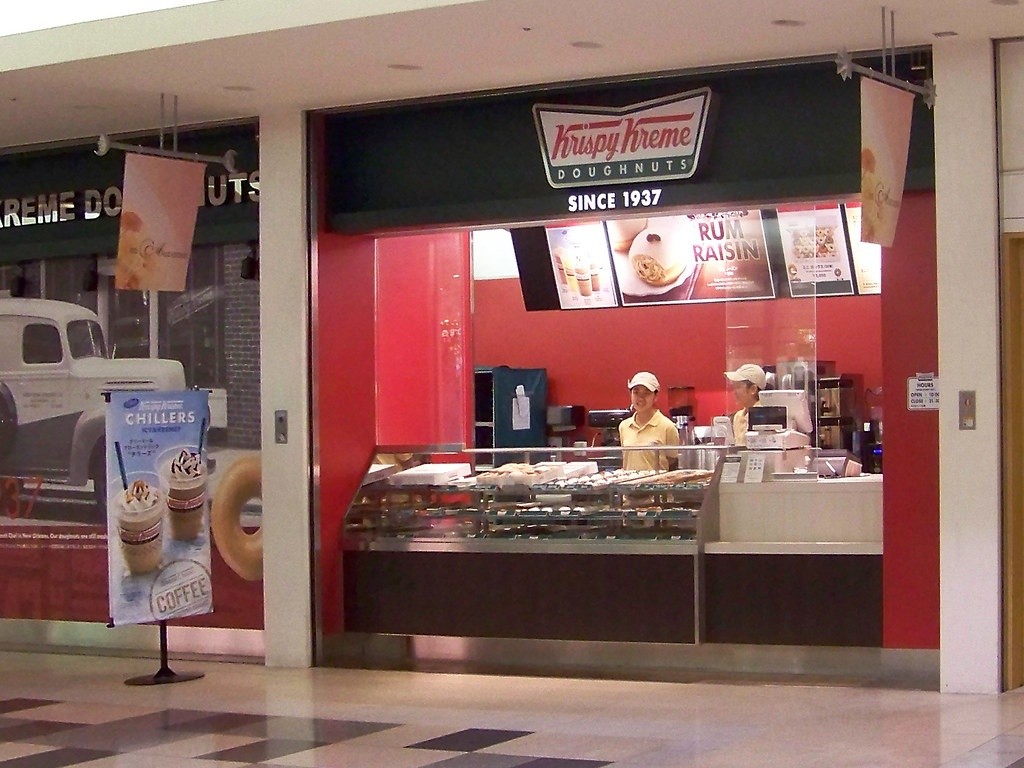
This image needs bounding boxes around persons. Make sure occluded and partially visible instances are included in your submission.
[724,364,767,446]
[619,371,680,511]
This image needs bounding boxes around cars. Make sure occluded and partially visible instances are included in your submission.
[0,297,229,516]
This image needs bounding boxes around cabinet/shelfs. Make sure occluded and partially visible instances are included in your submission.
[473,365,548,470]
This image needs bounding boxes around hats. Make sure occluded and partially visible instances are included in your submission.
[626,371,661,393]
[723,363,766,390]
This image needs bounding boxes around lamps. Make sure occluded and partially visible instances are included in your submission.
[239,241,260,280]
